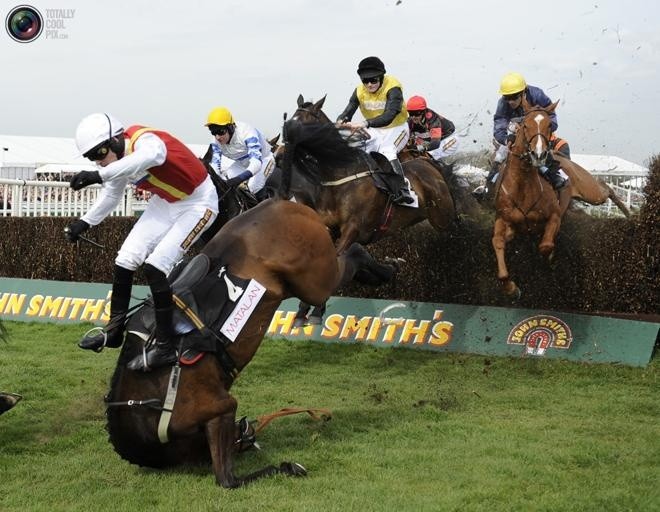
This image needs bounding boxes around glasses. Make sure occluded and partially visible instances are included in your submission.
[505,94,522,102]
[408,109,425,117]
[87,141,111,162]
[362,76,380,85]
[209,127,228,136]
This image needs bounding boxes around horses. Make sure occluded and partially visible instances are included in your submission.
[104,118,408,489]
[198,93,462,331]
[491,93,631,305]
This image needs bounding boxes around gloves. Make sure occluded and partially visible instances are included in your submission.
[62,218,89,244]
[227,176,242,189]
[69,168,101,192]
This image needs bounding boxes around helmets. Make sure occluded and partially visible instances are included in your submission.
[356,56,386,80]
[498,71,527,95]
[204,106,236,128]
[74,114,125,159]
[406,95,428,112]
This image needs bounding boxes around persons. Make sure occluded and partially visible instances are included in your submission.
[206,106,275,206]
[474,72,571,193]
[405,95,460,161]
[336,55,414,204]
[62,112,219,371]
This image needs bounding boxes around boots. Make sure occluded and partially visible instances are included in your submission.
[545,152,569,190]
[78,264,135,353]
[125,261,179,372]
[478,160,503,199]
[387,159,415,204]
